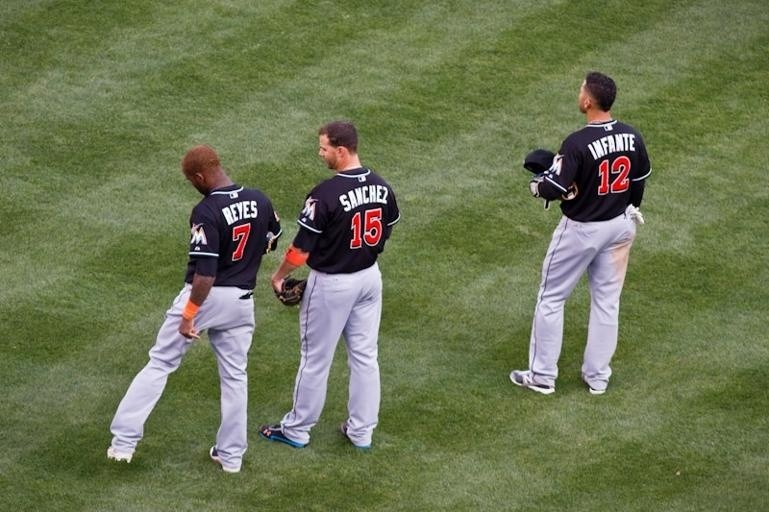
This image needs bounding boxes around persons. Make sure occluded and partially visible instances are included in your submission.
[106,146,284,473]
[507,73,651,395]
[271,122,400,448]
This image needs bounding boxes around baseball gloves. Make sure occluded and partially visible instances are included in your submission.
[275,275,307,306]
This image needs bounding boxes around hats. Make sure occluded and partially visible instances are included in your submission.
[524,149,556,172]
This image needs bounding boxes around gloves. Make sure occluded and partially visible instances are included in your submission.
[626,205,644,227]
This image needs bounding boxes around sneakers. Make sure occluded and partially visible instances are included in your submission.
[340,421,373,449]
[260,422,309,449]
[508,368,555,396]
[209,444,241,474]
[108,447,134,464]
[581,363,605,395]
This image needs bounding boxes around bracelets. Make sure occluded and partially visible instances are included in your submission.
[183,299,201,320]
